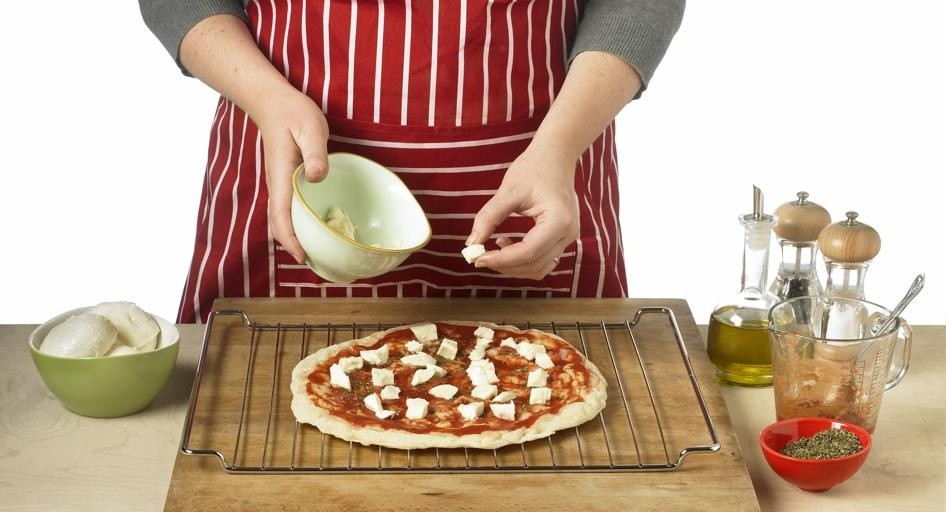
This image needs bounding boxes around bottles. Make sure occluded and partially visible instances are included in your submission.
[706,185,882,387]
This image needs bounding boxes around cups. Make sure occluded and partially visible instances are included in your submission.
[769,294,912,430]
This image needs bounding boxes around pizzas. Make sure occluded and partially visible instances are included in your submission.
[291,320,608,450]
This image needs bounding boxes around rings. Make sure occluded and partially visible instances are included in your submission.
[553,257,562,266]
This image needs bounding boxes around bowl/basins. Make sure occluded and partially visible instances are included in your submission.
[28,305,180,419]
[291,152,433,283]
[761,416,870,493]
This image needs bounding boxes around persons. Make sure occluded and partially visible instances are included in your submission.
[135,0,686,327]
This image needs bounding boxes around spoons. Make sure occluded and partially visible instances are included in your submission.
[818,274,927,418]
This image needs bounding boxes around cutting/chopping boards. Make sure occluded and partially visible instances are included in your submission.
[161,297,761,512]
